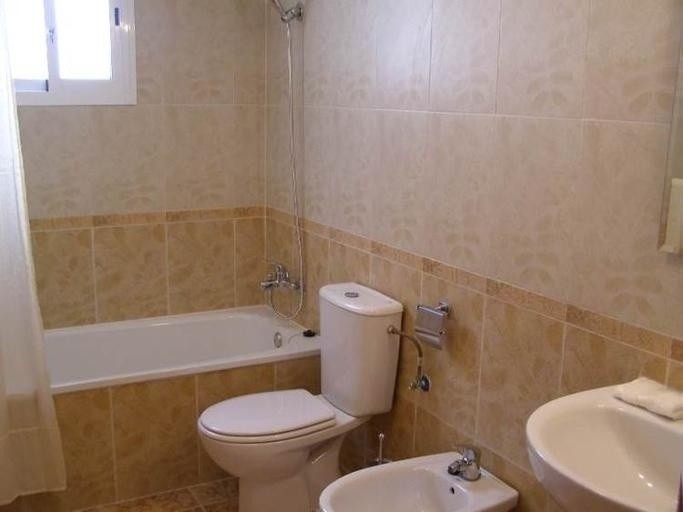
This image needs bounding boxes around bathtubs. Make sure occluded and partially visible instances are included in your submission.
[44,306,323,396]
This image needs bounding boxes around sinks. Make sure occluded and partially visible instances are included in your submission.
[318,451,520,511]
[525,384,683,512]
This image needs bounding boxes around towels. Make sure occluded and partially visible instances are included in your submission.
[610,375,683,421]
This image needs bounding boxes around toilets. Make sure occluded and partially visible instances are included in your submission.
[196,281,405,512]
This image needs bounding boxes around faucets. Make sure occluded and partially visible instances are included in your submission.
[447,441,481,479]
[260,257,298,292]
